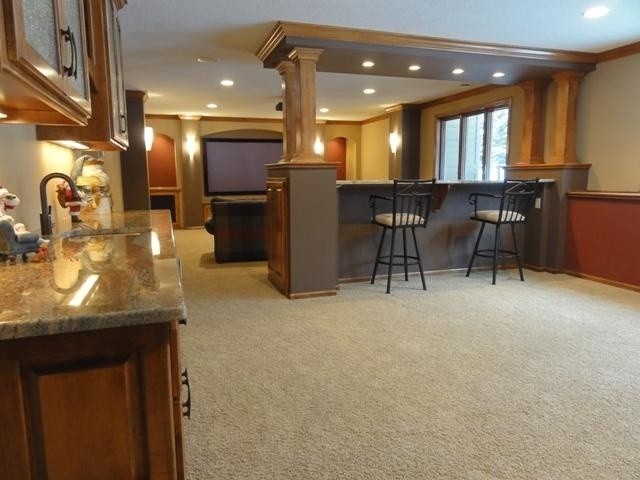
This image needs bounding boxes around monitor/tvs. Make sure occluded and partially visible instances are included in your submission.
[200,138,283,196]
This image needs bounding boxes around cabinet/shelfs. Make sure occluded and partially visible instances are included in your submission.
[2,0,135,152]
[0,323,197,480]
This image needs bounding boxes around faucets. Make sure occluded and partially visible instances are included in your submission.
[41,235,85,295]
[38,172,79,233]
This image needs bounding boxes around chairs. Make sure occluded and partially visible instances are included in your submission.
[372,178,435,294]
[464,187,533,283]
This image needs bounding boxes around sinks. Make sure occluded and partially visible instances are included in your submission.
[61,226,153,236]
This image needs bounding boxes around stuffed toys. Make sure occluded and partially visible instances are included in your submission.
[0,188,50,262]
[58,180,82,223]
